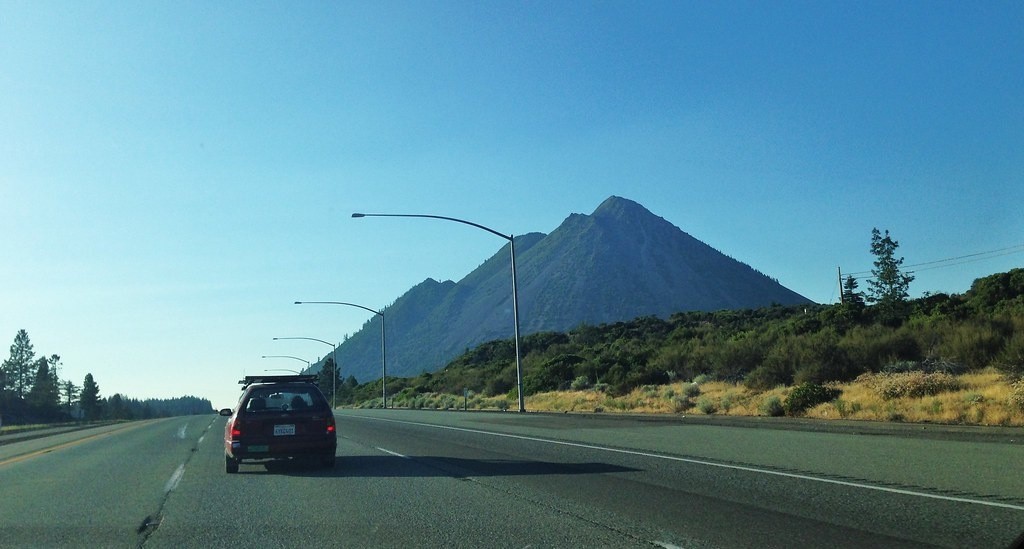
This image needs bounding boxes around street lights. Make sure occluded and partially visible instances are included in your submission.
[262,355,310,376]
[273,337,336,410]
[264,369,301,375]
[295,301,386,409]
[352,212,527,412]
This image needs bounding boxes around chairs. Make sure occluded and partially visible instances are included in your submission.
[250,399,266,412]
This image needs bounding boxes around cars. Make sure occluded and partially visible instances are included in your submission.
[219,382,337,475]
